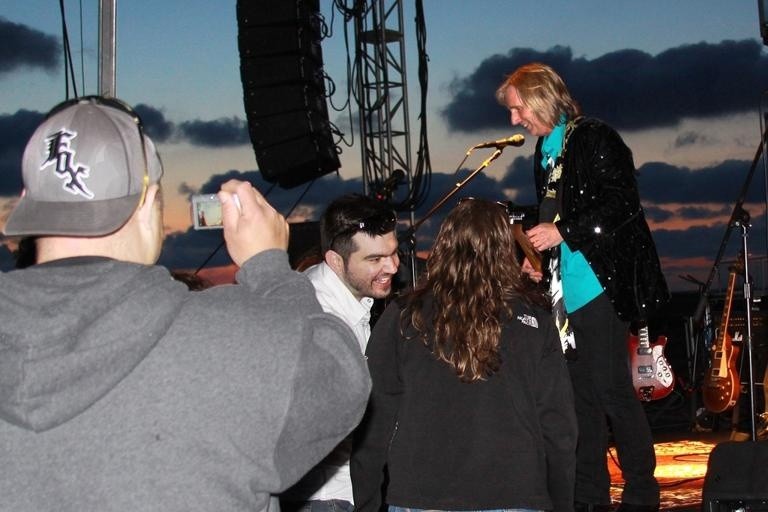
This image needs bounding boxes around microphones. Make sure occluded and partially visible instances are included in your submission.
[376,169,405,200]
[475,134,526,149]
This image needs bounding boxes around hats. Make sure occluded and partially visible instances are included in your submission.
[4,101,163,239]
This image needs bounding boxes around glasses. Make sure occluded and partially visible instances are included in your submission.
[328,209,401,250]
[458,197,509,215]
[44,94,150,210]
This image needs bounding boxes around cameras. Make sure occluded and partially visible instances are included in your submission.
[191,193,244,231]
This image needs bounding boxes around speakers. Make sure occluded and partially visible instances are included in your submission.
[701,440,768,512]
[235,0,342,191]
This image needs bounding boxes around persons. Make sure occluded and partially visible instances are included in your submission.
[1,95,373,512]
[274,199,400,512]
[340,205,579,511]
[494,64,667,510]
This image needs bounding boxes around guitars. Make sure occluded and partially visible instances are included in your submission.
[627,326,675,402]
[701,265,740,413]
[497,199,553,314]
